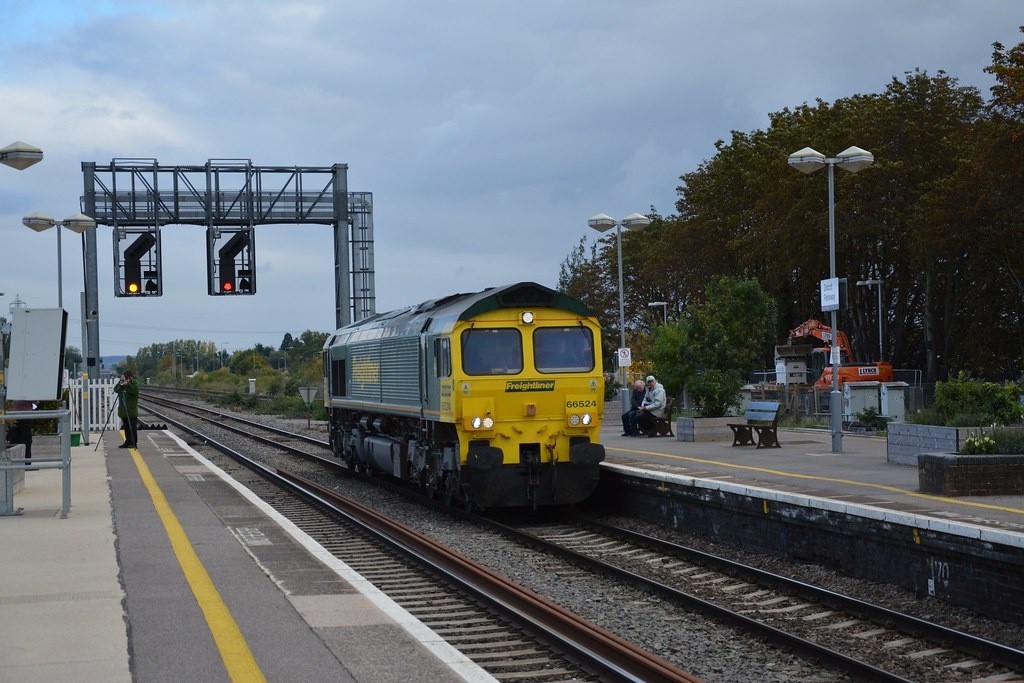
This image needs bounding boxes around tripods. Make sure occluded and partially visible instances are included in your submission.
[94,387,139,451]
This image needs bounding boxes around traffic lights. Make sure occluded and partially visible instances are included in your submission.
[123,259,142,294]
[218,258,237,294]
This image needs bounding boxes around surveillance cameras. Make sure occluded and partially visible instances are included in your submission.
[90,311,97,318]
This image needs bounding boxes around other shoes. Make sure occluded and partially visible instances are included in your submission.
[119,442,137,448]
[620,431,638,436]
[647,426,656,436]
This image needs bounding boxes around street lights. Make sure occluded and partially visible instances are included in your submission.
[23,211,96,308]
[787,145,875,453]
[588,212,653,415]
[1,140,46,171]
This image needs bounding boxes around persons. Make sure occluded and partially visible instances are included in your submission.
[113,369,139,449]
[628,375,666,437]
[544,334,580,367]
[620,380,646,438]
[5,399,40,471]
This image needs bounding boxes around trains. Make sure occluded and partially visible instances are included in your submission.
[320,280,606,508]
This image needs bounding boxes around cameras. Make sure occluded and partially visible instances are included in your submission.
[118,380,125,387]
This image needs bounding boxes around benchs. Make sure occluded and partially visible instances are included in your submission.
[649,398,676,437]
[725,400,782,449]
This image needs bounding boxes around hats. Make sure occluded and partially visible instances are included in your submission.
[124,369,132,376]
[646,375,656,381]
